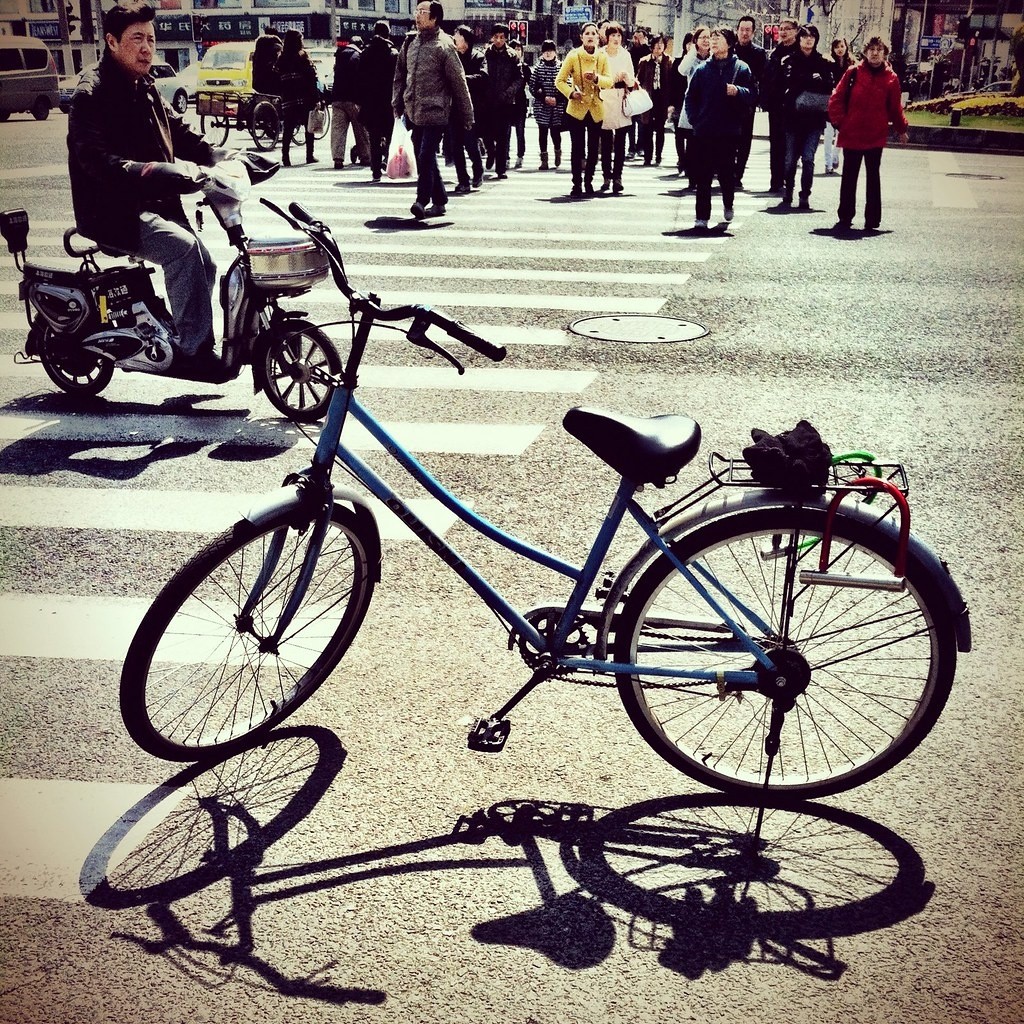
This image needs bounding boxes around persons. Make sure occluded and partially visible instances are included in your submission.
[249,18,543,193]
[65,2,236,384]
[531,14,932,212]
[272,28,322,167]
[827,36,909,235]
[251,25,284,98]
[554,20,614,199]
[391,0,475,219]
[598,22,635,192]
[683,27,754,228]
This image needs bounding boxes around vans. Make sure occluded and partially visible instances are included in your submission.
[198,40,257,99]
[0,34,60,124]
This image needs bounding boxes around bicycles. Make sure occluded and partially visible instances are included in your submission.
[264,102,330,141]
[119,196,973,842]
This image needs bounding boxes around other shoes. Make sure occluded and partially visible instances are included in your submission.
[411,202,426,219]
[485,152,494,169]
[454,182,470,194]
[282,154,291,167]
[514,156,523,168]
[425,205,446,216]
[473,167,483,187]
[306,156,318,164]
[570,147,879,236]
[506,160,511,170]
[334,160,344,169]
[176,351,238,383]
[498,171,507,179]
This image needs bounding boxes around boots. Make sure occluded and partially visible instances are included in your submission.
[538,153,548,169]
[554,148,561,166]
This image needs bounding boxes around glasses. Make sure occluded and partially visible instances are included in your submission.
[412,9,430,16]
[778,26,796,33]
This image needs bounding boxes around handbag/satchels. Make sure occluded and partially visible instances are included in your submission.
[796,92,833,122]
[387,116,416,179]
[308,104,324,133]
[622,76,653,117]
[831,65,858,130]
[729,119,754,152]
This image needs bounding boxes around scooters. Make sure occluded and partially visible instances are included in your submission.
[1,144,345,422]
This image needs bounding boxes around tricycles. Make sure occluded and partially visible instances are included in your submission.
[195,82,311,151]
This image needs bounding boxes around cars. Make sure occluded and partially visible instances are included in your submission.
[950,81,1012,96]
[57,58,189,114]
[176,61,201,95]
[306,47,336,95]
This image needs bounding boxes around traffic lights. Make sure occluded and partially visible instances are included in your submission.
[510,20,528,48]
